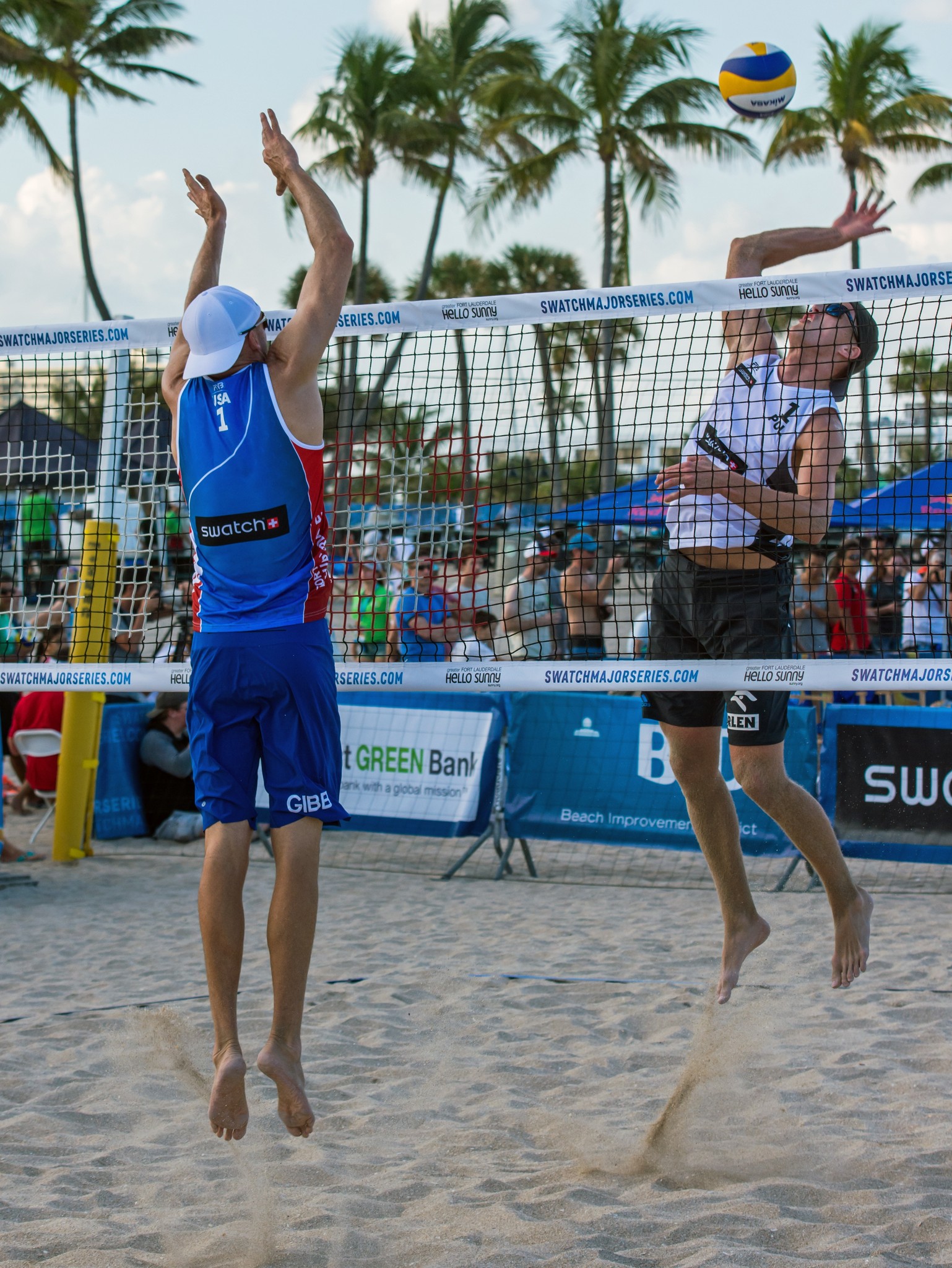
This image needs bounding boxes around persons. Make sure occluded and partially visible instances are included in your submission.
[644,181,880,1006]
[161,107,355,1143]
[0,485,952,844]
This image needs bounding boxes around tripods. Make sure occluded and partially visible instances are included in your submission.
[152,615,194,663]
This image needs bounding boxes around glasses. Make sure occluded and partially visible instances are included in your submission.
[824,303,859,343]
[0,588,15,594]
[848,554,860,561]
[239,311,269,335]
[410,564,430,571]
[538,557,549,562]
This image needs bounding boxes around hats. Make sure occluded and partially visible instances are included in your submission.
[831,301,879,403]
[363,530,383,557]
[921,538,938,557]
[182,284,262,380]
[524,540,558,558]
[147,692,191,719]
[534,526,565,541]
[57,566,80,593]
[357,557,382,571]
[567,532,603,552]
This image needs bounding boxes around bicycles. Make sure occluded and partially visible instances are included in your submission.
[631,544,671,597]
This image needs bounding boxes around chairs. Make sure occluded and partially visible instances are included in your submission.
[13,730,61,845]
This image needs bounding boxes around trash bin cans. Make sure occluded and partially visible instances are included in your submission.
[494,536,533,597]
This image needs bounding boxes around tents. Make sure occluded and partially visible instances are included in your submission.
[0,399,180,609]
[550,460,952,536]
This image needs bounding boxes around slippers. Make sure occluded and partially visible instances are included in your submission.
[0,851,46,863]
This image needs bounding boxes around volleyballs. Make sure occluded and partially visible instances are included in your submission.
[718,41,797,119]
